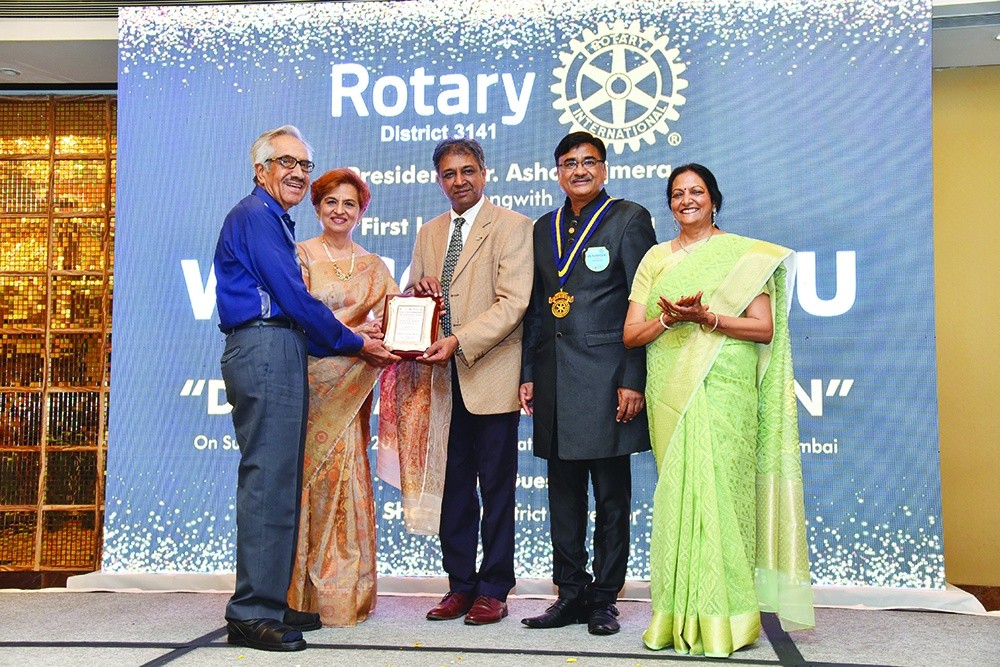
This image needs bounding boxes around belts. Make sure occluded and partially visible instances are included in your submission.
[224,317,307,334]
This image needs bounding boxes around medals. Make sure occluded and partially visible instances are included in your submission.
[547,291,574,317]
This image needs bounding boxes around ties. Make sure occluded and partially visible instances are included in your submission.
[440,218,466,337]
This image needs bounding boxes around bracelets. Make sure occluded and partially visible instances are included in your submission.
[700,311,718,336]
[659,313,677,331]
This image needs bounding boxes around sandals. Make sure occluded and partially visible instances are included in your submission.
[226,619,307,652]
[283,608,323,632]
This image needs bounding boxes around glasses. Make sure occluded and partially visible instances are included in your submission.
[557,157,602,169]
[266,155,315,173]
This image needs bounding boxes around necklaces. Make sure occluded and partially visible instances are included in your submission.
[676,226,716,254]
[317,236,356,280]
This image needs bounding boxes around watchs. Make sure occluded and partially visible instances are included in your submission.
[456,343,462,355]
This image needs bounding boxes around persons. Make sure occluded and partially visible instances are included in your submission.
[297,166,400,628]
[405,138,535,626]
[214,123,403,652]
[626,164,816,658]
[518,131,658,636]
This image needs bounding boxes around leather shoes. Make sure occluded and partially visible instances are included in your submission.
[587,603,620,634]
[426,591,472,620]
[464,595,508,625]
[521,600,587,628]
